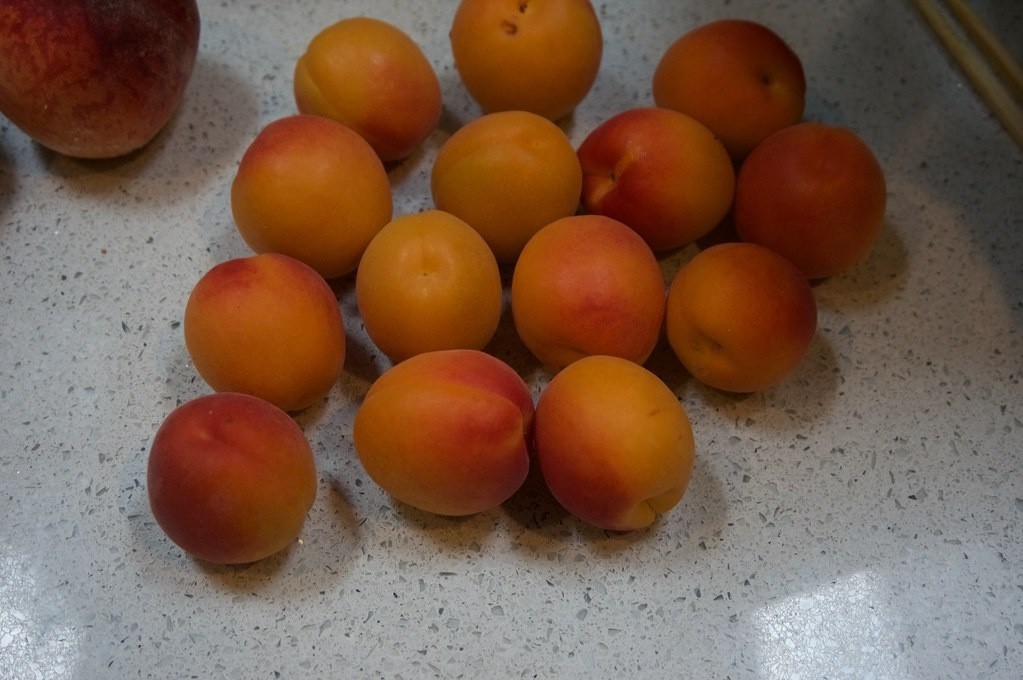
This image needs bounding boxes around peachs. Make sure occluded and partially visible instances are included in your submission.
[0,0,203,163]
